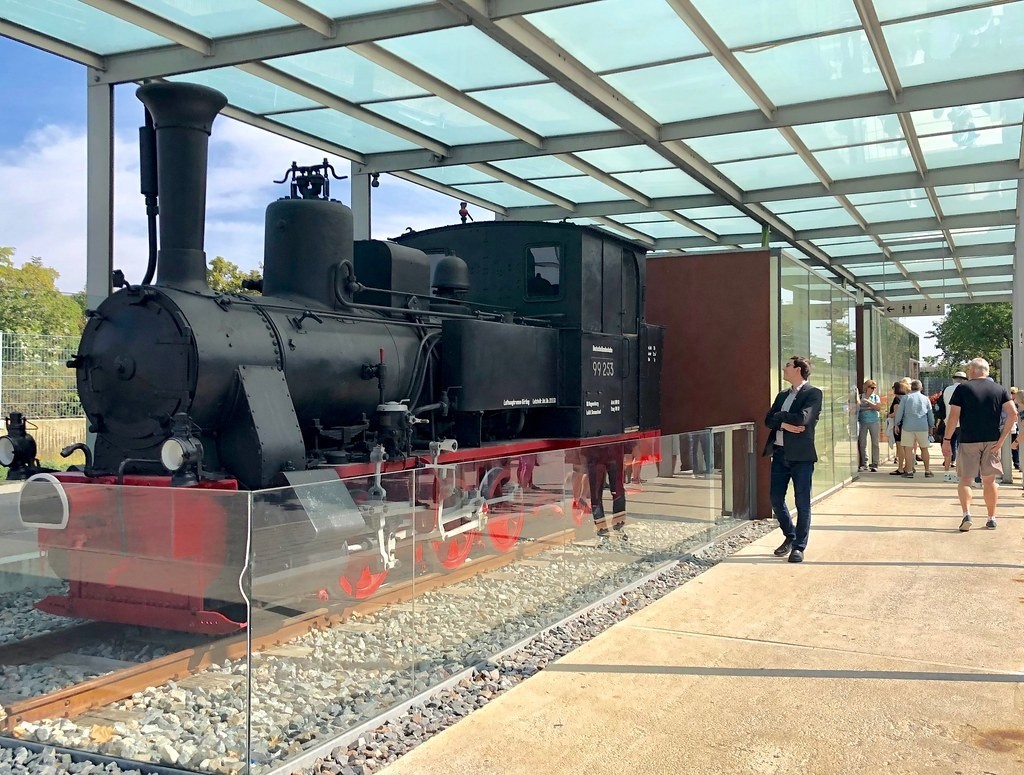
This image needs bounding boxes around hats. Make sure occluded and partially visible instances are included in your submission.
[902,377,912,383]
[952,371,969,381]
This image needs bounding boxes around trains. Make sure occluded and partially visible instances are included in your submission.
[0,83,667,640]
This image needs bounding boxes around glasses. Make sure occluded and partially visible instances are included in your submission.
[785,362,795,367]
[1011,392,1015,394]
[892,386,895,389]
[868,387,877,389]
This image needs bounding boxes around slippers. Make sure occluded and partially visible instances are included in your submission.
[889,470,905,475]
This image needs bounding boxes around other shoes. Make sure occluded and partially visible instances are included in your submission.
[951,464,956,467]
[572,501,580,509]
[860,465,869,470]
[871,468,878,472]
[894,458,899,464]
[902,472,914,478]
[577,498,591,513]
[973,482,982,488]
[925,471,934,477]
[495,502,510,508]
[943,461,945,466]
[986,520,997,529]
[529,484,539,490]
[943,474,960,482]
[632,477,647,484]
[959,515,973,530]
[916,455,923,461]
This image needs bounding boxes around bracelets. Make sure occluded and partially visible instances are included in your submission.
[1015,440,1018,443]
[943,438,951,441]
[887,414,888,418]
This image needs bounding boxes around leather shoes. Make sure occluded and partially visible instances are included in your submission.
[789,549,803,562]
[774,538,793,556]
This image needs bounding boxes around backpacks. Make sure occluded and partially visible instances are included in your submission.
[934,385,949,420]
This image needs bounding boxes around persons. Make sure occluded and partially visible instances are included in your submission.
[762,355,823,563]
[859,380,881,472]
[941,357,1018,531]
[406,430,714,546]
[883,377,934,478]
[930,371,1024,497]
